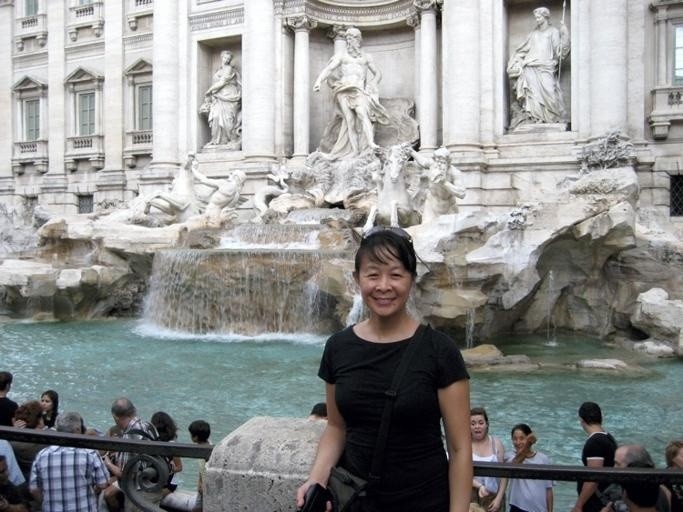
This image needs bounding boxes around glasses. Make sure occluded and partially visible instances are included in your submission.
[360,226,414,252]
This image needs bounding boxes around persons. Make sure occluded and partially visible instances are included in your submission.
[190,160,246,229]
[308,403,328,420]
[296,224,473,512]
[405,146,466,226]
[469,407,508,512]
[504,424,553,512]
[312,28,391,161]
[189,420,213,512]
[505,7,572,133]
[570,402,683,512]
[198,51,242,150]
[0,371,183,512]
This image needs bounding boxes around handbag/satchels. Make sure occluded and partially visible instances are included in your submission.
[326,465,373,512]
